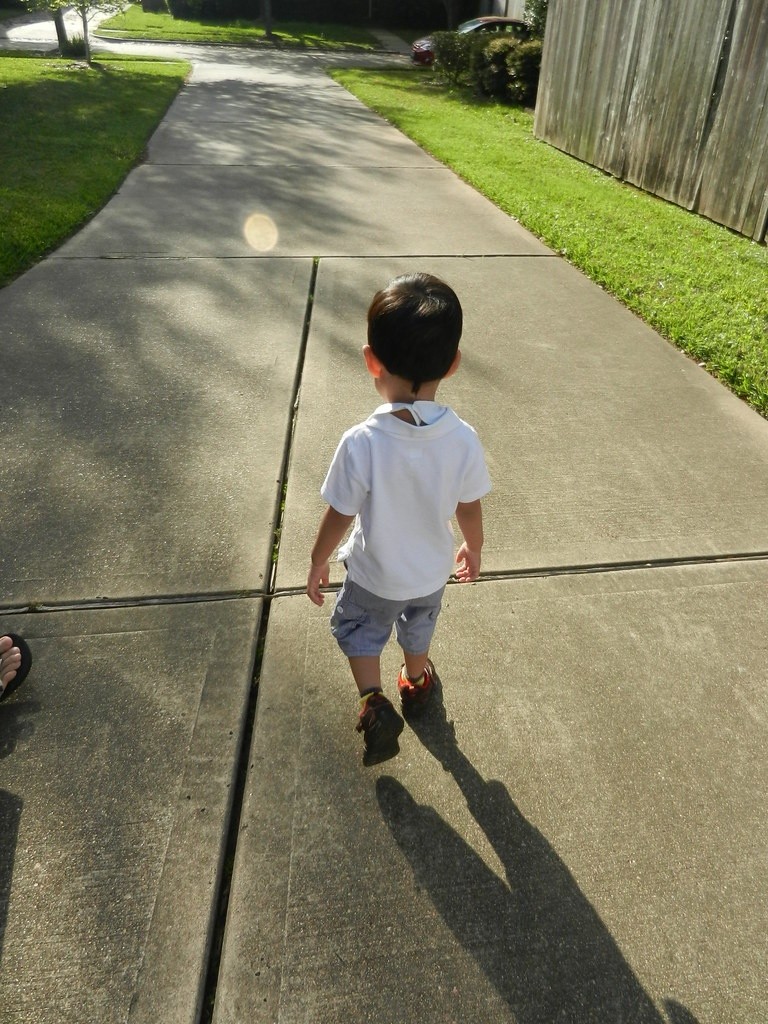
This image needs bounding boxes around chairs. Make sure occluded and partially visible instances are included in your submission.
[485,26,497,33]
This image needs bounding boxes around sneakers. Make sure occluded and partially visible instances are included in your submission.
[397,659,435,711]
[357,695,405,767]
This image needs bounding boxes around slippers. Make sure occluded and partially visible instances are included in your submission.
[0,633,32,702]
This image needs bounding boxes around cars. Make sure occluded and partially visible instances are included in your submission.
[409,17,531,71]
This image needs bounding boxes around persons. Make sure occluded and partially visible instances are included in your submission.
[306,275,493,766]
